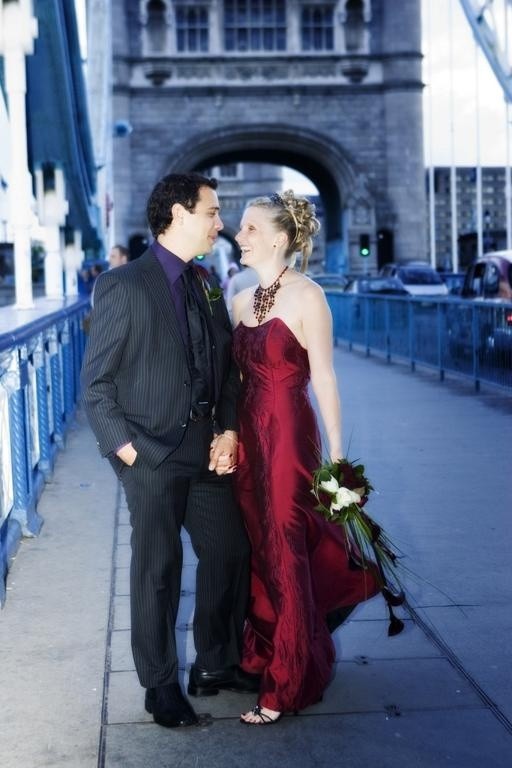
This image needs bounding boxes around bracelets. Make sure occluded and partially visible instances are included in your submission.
[210,435,220,450]
[221,434,238,446]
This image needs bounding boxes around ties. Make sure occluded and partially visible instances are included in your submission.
[181,269,209,396]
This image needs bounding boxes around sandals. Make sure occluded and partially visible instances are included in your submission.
[239,702,285,725]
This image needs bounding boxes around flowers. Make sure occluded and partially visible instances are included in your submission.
[304,427,411,637]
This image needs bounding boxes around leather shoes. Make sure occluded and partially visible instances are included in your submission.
[188,665,259,698]
[143,685,198,729]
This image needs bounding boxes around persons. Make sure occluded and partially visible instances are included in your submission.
[78,172,279,730]
[128,233,150,260]
[89,264,103,277]
[224,267,237,302]
[81,269,91,283]
[90,245,129,310]
[209,266,222,286]
[208,186,386,729]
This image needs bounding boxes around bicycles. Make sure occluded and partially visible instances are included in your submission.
[379,262,447,314]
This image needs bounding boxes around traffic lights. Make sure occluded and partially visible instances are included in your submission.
[359,233,370,257]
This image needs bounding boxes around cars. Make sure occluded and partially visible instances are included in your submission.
[441,250,511,369]
[342,274,412,299]
[306,273,349,294]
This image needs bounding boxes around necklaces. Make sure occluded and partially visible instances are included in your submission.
[252,265,290,325]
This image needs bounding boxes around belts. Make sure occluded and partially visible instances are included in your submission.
[186,406,212,423]
